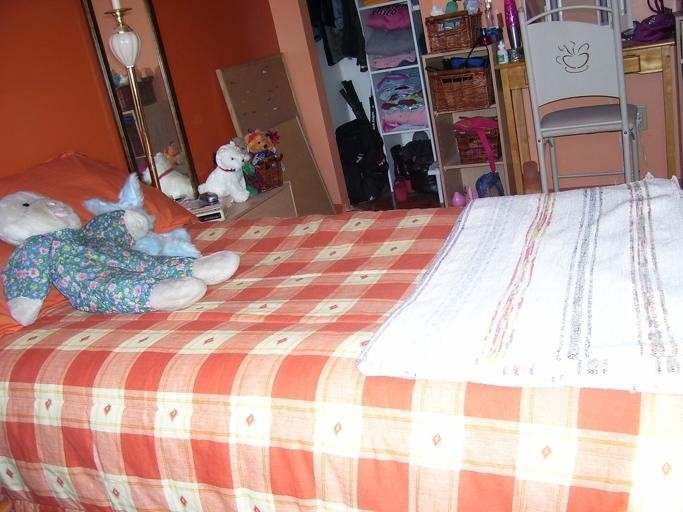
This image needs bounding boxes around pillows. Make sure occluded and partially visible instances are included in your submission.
[1,149,194,336]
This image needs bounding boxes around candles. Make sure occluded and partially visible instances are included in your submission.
[111,1,120,10]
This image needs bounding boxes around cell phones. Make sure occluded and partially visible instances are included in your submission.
[199,191,218,203]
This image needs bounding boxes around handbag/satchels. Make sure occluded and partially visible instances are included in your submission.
[632,0,675,43]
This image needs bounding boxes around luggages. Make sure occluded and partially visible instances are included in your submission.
[334,117,388,207]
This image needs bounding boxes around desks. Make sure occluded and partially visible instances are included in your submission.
[493,41,679,195]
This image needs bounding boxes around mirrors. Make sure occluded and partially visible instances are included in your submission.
[83,1,198,201]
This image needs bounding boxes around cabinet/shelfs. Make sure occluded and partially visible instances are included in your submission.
[304,0,514,207]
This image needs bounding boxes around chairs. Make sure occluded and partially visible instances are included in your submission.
[517,4,637,194]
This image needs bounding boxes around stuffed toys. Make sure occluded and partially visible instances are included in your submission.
[0,189,241,327]
[140,152,192,200]
[195,140,248,203]
[229,128,282,195]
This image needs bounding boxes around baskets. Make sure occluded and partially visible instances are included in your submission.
[424,9,483,54]
[452,116,502,164]
[243,153,284,193]
[428,65,494,112]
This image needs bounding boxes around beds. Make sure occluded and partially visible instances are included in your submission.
[0,211,681,510]
